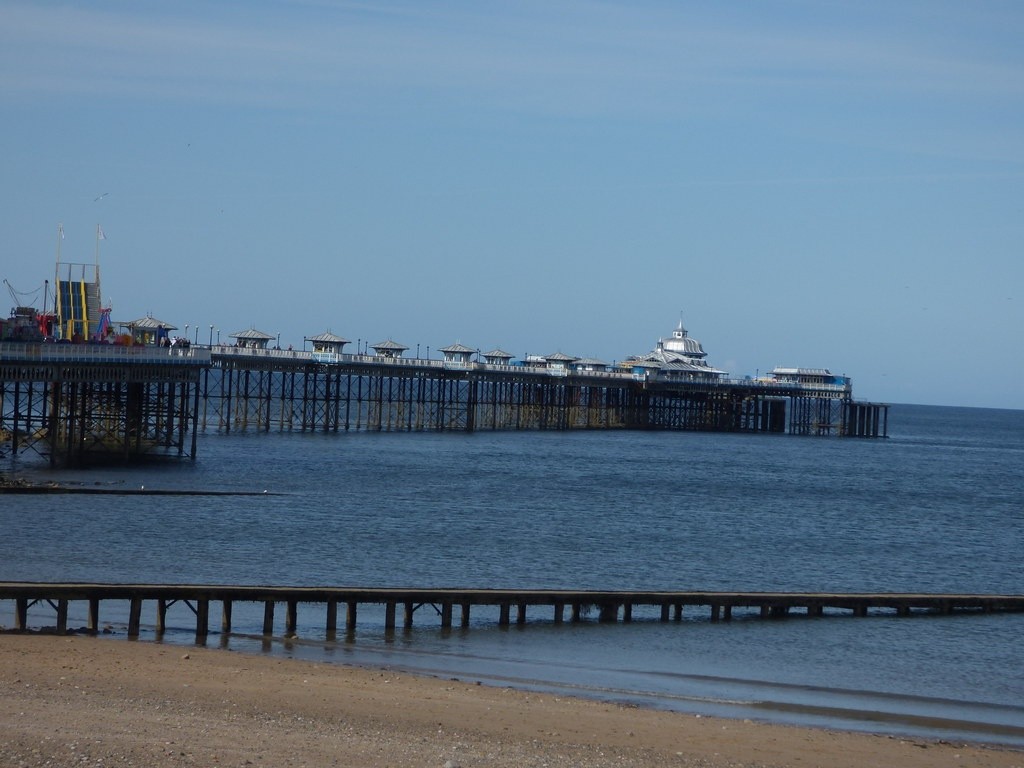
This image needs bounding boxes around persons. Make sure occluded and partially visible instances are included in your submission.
[161,335,191,356]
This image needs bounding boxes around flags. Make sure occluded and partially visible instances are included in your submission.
[99,225,106,239]
[60,225,65,238]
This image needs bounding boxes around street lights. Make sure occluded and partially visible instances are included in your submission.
[477,348,481,363]
[365,341,368,355]
[304,336,307,351]
[216,329,221,344]
[195,326,199,344]
[277,332,281,349]
[426,345,429,360]
[210,325,214,345]
[524,353,532,366]
[358,339,360,355]
[416,343,419,359]
[184,324,189,337]
